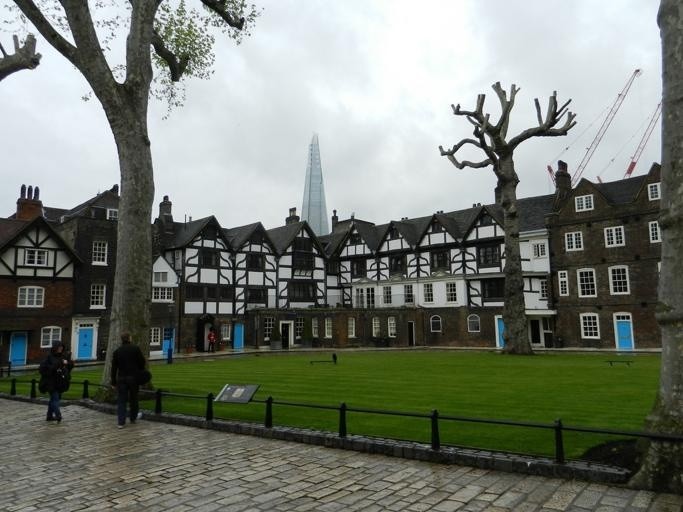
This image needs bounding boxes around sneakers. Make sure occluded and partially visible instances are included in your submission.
[130,412,143,423]
[118,426,124,429]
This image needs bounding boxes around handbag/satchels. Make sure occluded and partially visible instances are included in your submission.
[138,367,151,385]
[57,367,71,385]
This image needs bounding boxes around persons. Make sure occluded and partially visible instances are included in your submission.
[110,333,146,429]
[37,341,74,422]
[207,326,216,353]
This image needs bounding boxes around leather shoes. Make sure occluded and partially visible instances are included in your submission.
[47,417,55,421]
[55,416,61,421]
